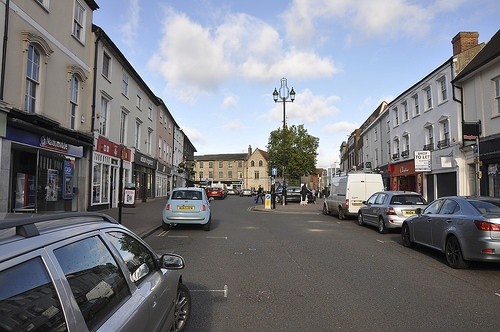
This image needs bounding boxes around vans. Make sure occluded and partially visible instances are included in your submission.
[276,186,311,192]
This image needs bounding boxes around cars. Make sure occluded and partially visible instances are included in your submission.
[228,189,239,195]
[357,191,428,234]
[271,187,316,204]
[401,196,500,269]
[223,190,227,194]
[239,189,252,197]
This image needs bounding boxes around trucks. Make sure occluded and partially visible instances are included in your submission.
[211,183,228,197]
[323,173,384,220]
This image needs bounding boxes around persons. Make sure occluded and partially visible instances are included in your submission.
[300,183,308,205]
[255,185,265,205]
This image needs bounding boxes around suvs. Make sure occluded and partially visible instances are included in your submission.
[0,212,191,332]
[162,187,215,231]
[206,187,225,200]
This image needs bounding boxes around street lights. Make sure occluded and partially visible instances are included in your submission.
[273,77,296,205]
